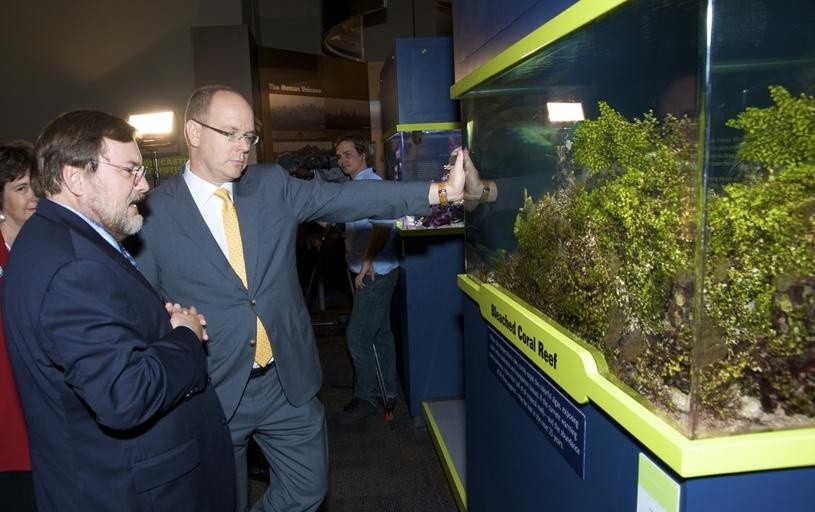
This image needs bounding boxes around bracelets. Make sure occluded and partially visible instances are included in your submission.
[437,182,448,206]
[479,179,491,204]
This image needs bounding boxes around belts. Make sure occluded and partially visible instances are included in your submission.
[247,362,276,378]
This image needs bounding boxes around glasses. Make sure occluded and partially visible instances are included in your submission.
[93,160,148,187]
[192,118,259,145]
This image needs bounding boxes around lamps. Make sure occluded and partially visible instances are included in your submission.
[126,109,182,179]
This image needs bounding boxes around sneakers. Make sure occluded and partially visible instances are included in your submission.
[378,395,397,410]
[248,463,269,481]
[343,397,377,415]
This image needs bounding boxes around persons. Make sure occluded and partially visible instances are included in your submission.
[122,84,466,510]
[1,107,239,511]
[0,137,41,511]
[464,148,559,213]
[332,132,402,415]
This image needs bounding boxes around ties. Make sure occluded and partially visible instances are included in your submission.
[214,188,273,369]
